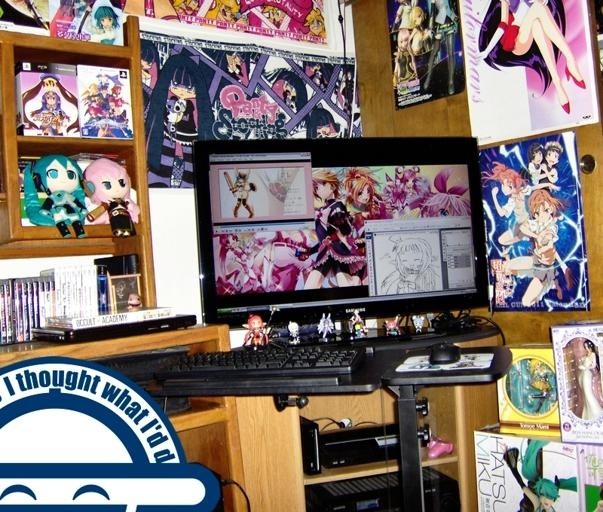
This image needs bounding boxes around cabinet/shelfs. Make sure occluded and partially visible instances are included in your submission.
[0,15,158,309]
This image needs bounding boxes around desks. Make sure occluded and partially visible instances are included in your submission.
[1,323,249,511]
[233,325,504,512]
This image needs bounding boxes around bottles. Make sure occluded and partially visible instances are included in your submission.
[97,266,107,315]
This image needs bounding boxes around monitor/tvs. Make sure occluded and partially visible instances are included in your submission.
[190,136,490,327]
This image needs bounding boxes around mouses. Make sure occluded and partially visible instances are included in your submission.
[430,342,460,363]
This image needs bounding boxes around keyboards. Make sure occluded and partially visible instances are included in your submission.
[149,348,366,378]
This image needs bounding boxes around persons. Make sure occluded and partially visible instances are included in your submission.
[577,340,601,420]
[240,304,425,348]
[21,152,142,239]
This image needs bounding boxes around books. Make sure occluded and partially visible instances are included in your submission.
[46,306,179,333]
[0,251,139,351]
